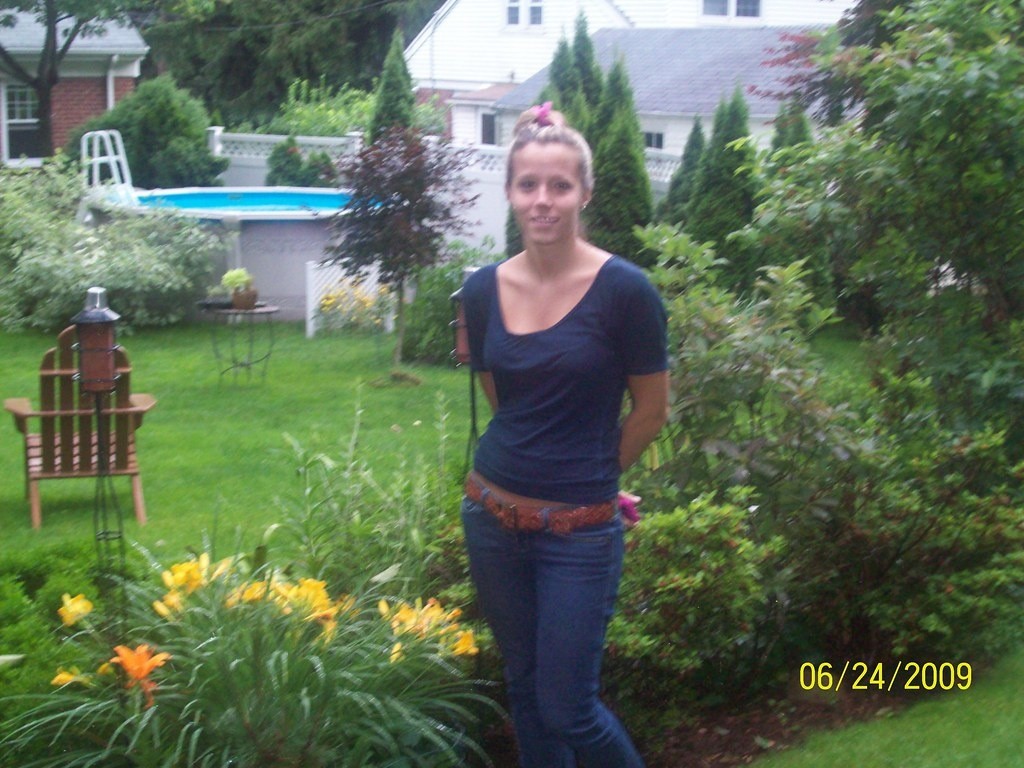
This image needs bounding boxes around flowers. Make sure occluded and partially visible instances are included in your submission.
[619,495,642,523]
[531,100,554,127]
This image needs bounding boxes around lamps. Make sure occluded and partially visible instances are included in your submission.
[72,286,121,687]
[450,267,481,486]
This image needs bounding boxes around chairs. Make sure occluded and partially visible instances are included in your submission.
[5,325,160,530]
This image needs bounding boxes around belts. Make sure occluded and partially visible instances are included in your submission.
[466,475,615,535]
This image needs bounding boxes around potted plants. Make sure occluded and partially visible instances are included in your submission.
[221,266,258,311]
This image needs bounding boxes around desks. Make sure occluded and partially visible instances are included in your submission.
[202,306,280,393]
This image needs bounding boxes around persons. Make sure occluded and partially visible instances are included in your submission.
[459,105,666,768]
